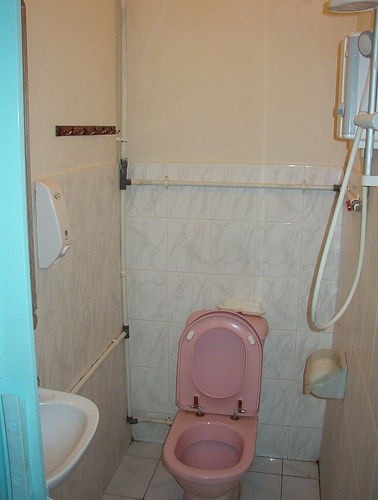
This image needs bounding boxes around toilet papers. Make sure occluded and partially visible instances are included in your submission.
[303,353,336,395]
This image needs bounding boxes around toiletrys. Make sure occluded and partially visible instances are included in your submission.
[35,178,71,270]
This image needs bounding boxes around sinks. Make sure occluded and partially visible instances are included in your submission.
[38,387,99,491]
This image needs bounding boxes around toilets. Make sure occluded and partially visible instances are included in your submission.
[162,311,270,499]
